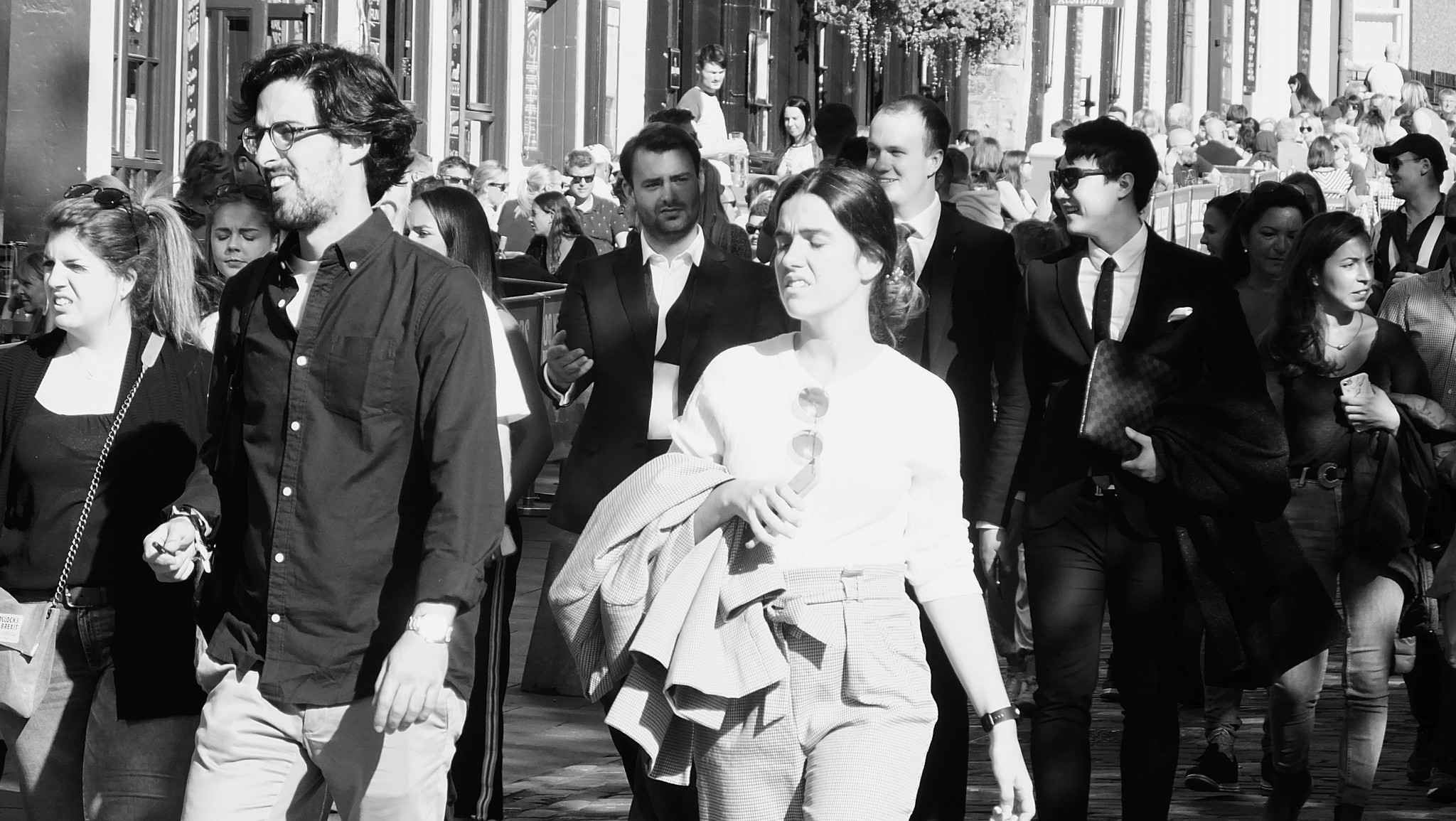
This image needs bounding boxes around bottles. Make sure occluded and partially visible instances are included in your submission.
[495,235,508,259]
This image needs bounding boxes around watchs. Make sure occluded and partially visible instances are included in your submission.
[405,612,454,644]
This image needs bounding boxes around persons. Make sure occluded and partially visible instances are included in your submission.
[1,171,214,821]
[1,41,509,821]
[974,117,1291,820]
[666,165,1036,821]
[676,43,750,187]
[171,44,1456,821]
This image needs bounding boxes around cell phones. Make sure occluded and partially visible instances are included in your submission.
[1340,372,1375,433]
[744,458,822,549]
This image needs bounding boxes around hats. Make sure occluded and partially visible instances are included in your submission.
[1373,133,1448,180]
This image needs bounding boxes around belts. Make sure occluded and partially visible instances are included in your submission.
[1293,463,1346,482]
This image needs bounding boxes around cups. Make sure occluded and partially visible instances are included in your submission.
[729,131,744,155]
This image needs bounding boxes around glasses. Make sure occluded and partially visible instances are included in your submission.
[1444,217,1456,234]
[1023,160,1031,165]
[1252,182,1306,198]
[216,183,270,200]
[64,184,140,254]
[442,175,469,185]
[1172,141,1195,148]
[1300,126,1312,132]
[1049,167,1106,190]
[571,175,595,183]
[1388,156,1425,172]
[1334,145,1340,152]
[488,183,507,191]
[237,121,328,151]
[1352,106,1356,110]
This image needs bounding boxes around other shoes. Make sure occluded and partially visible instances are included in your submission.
[1407,742,1432,782]
[1431,759,1456,803]
[1259,771,1276,789]
[1184,742,1239,792]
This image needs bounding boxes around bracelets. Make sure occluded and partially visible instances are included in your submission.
[980,706,1022,733]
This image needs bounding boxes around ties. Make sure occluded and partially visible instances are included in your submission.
[1092,257,1116,340]
[894,224,916,284]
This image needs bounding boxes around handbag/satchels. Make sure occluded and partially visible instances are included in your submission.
[1080,338,1171,458]
[0,598,60,718]
